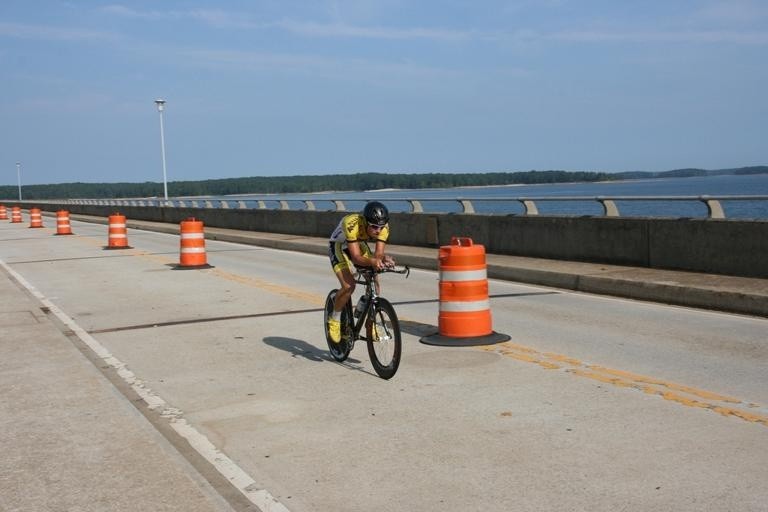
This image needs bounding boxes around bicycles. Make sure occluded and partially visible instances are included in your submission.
[323,266,412,380]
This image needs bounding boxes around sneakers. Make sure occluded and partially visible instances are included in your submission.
[329,312,341,343]
[372,323,378,342]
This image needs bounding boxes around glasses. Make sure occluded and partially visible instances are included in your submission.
[372,225,384,230]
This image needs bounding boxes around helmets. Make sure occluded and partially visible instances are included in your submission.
[363,202,389,225]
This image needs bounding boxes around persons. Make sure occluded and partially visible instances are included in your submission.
[328,201,395,344]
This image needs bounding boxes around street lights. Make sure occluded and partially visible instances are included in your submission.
[154,99,170,200]
[16,162,22,201]
